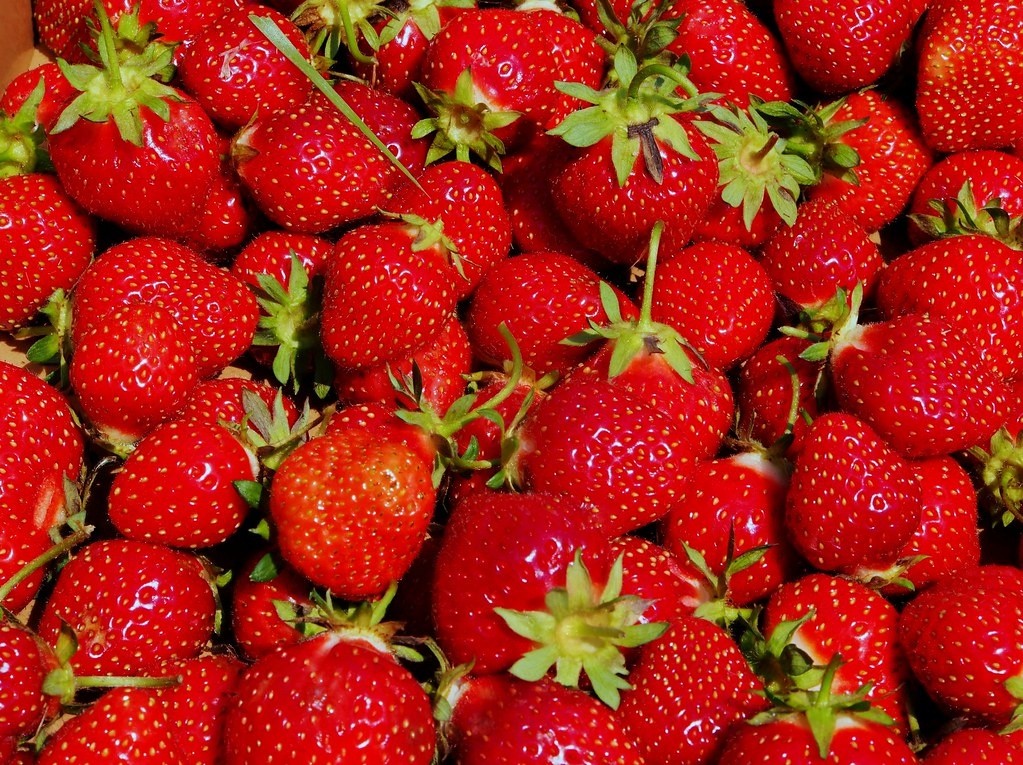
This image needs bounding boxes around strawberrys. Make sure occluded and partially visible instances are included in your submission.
[0,0,1023,765]
